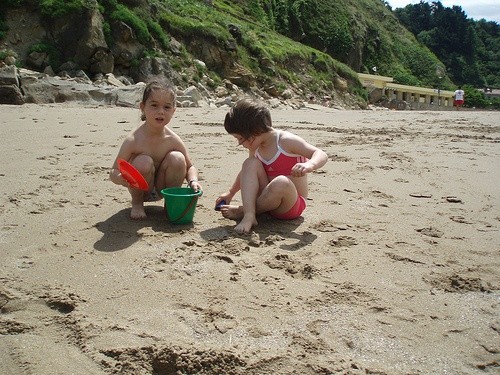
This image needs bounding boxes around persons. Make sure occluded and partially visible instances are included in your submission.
[216,99,328,236]
[109,80,203,219]
[452,86,464,111]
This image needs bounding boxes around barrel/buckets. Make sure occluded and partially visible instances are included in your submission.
[160,186,203,224]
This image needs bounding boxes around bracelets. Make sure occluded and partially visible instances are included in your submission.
[187,179,197,188]
